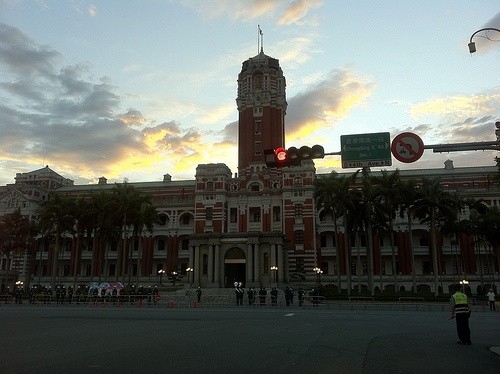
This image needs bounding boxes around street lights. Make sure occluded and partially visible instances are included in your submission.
[15,280,24,290]
[459,279,469,293]
[171,271,177,286]
[158,269,165,284]
[312,267,323,296]
[270,264,278,286]
[186,267,193,283]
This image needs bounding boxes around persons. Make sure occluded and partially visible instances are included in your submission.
[487,289,496,312]
[452,283,474,346]
[195,286,202,303]
[3,283,161,307]
[234,286,322,309]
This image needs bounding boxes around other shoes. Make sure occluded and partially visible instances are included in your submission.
[465,342,473,345]
[457,340,465,345]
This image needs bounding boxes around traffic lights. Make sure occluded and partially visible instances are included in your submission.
[263,145,324,167]
